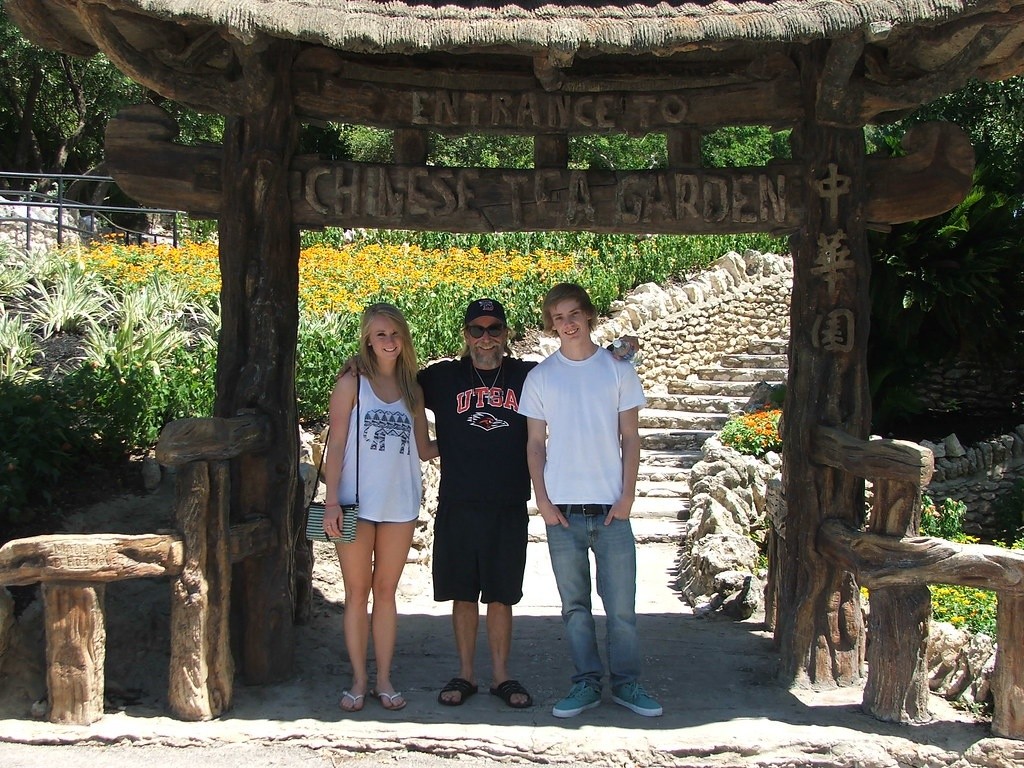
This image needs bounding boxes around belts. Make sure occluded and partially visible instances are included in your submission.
[556,504,613,518]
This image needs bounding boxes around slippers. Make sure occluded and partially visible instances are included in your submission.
[340,690,365,711]
[490,680,532,708]
[370,689,407,710]
[438,677,479,706]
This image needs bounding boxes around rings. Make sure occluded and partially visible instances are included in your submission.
[326,523,331,528]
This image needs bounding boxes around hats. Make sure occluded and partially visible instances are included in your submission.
[465,299,506,326]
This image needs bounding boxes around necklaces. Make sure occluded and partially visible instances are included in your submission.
[473,363,502,404]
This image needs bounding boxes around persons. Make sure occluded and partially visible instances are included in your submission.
[322,303,440,712]
[517,284,663,716]
[336,295,641,709]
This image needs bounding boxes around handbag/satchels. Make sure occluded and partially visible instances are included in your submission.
[305,501,358,543]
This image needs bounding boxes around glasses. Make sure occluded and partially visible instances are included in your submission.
[466,325,506,338]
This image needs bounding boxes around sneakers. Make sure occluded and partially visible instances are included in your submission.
[553,680,601,718]
[610,683,663,716]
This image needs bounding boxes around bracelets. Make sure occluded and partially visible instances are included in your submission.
[325,501,340,507]
